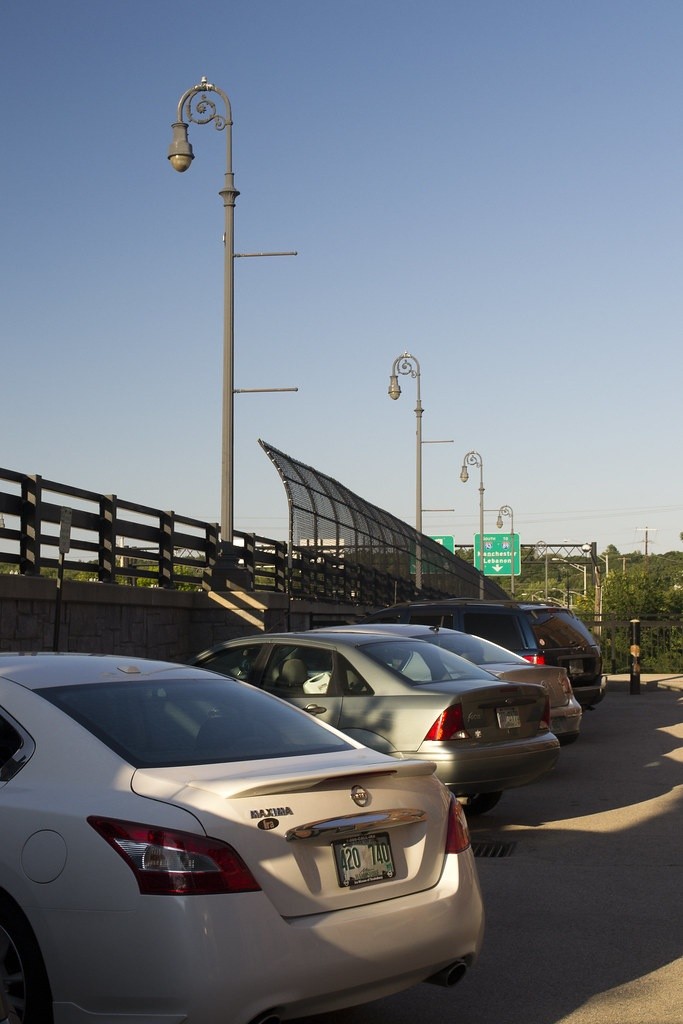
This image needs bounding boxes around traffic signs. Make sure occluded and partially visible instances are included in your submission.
[474,533,521,577]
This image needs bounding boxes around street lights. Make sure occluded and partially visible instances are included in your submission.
[552,558,587,596]
[460,451,504,600]
[496,505,515,600]
[582,544,601,635]
[533,541,547,601]
[388,349,454,594]
[166,75,298,590]
[552,588,572,605]
[532,591,544,602]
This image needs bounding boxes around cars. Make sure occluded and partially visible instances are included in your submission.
[147,632,562,817]
[0,651,485,1023]
[306,624,582,747]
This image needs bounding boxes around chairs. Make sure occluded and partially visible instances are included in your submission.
[273,659,308,697]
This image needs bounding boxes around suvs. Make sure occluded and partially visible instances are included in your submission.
[357,598,608,713]
